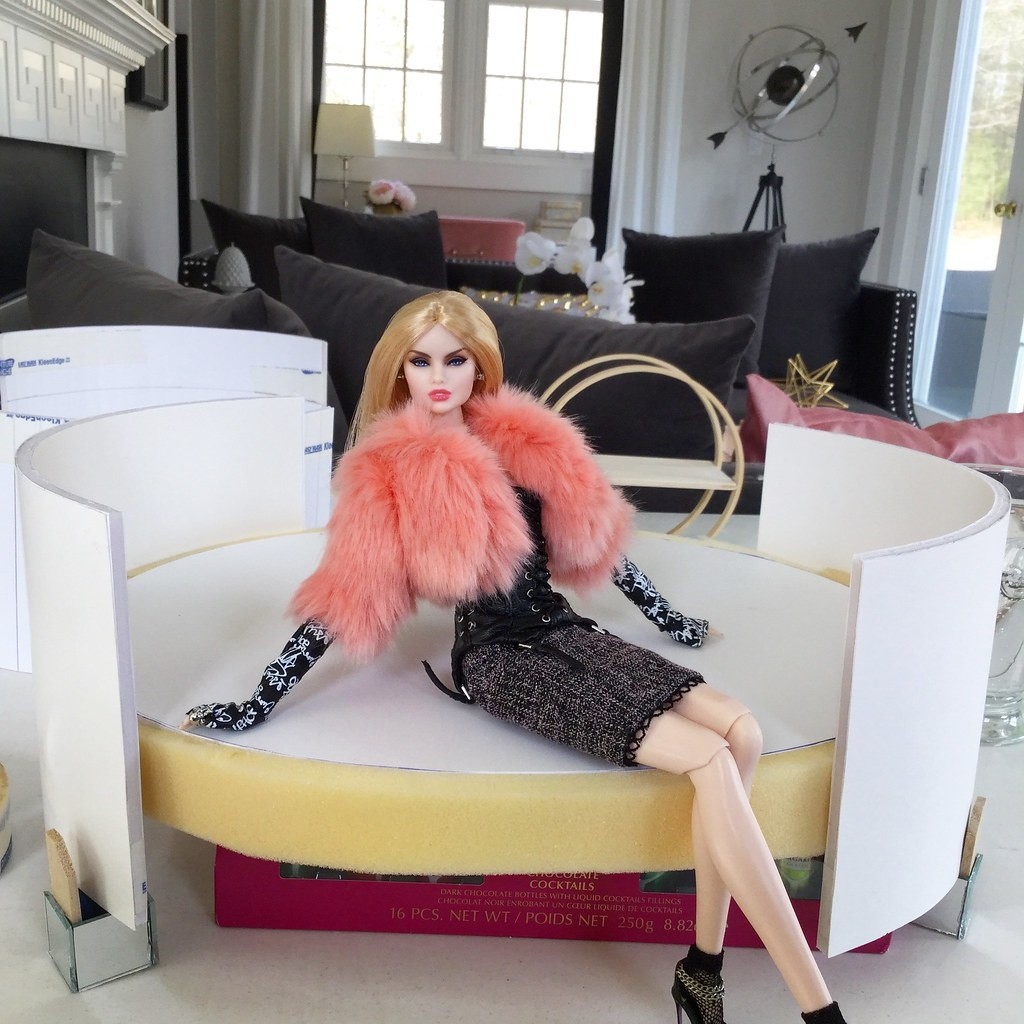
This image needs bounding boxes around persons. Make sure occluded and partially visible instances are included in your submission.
[180,292,847,1024]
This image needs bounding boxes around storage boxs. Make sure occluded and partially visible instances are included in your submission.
[211,844,894,957]
[40,888,159,995]
[908,854,984,940]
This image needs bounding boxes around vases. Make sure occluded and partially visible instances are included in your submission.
[372,203,401,216]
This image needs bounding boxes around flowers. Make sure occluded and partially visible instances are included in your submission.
[362,178,418,212]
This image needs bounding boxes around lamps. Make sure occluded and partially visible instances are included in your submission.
[313,102,378,208]
[211,241,257,295]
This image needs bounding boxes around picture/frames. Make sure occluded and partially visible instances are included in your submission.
[125,0,171,110]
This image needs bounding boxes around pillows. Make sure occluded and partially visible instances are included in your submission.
[300,195,449,292]
[273,239,755,515]
[758,227,883,388]
[621,225,784,388]
[24,228,349,453]
[200,199,316,302]
